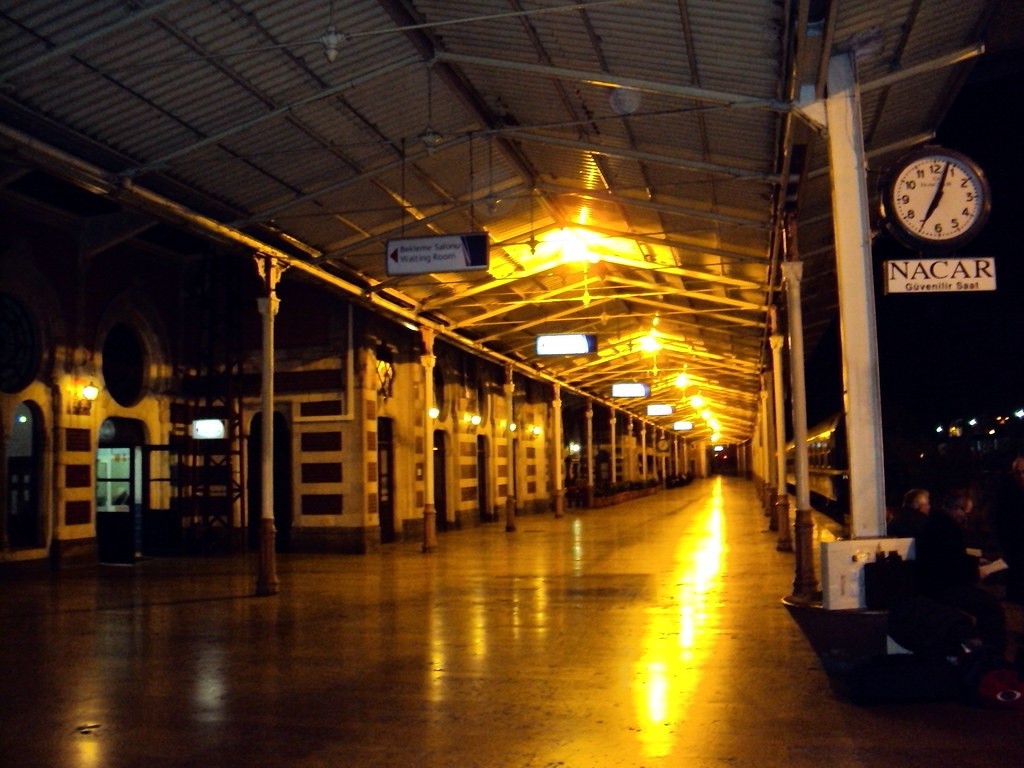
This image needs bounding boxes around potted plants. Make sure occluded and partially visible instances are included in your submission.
[578,478,663,507]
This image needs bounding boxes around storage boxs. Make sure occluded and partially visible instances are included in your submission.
[820,536,915,609]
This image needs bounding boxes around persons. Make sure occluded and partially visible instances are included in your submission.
[666,472,692,488]
[888,456,1024,671]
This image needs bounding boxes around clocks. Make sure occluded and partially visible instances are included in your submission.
[874,143,992,255]
